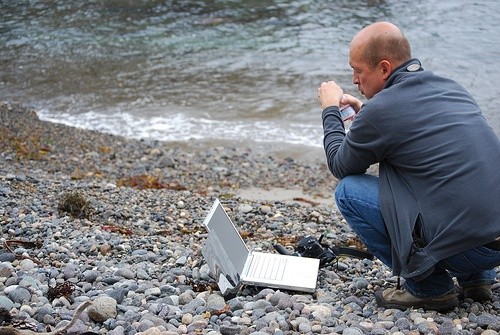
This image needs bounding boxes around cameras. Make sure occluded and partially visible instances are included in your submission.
[292,235,336,266]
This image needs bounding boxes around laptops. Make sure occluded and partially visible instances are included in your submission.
[203,199,320,292]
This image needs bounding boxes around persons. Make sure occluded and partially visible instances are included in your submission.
[317,21,500,311]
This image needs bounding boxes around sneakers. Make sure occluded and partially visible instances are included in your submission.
[374,284,460,314]
[462,283,493,301]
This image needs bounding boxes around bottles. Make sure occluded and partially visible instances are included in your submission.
[338,99,357,134]
